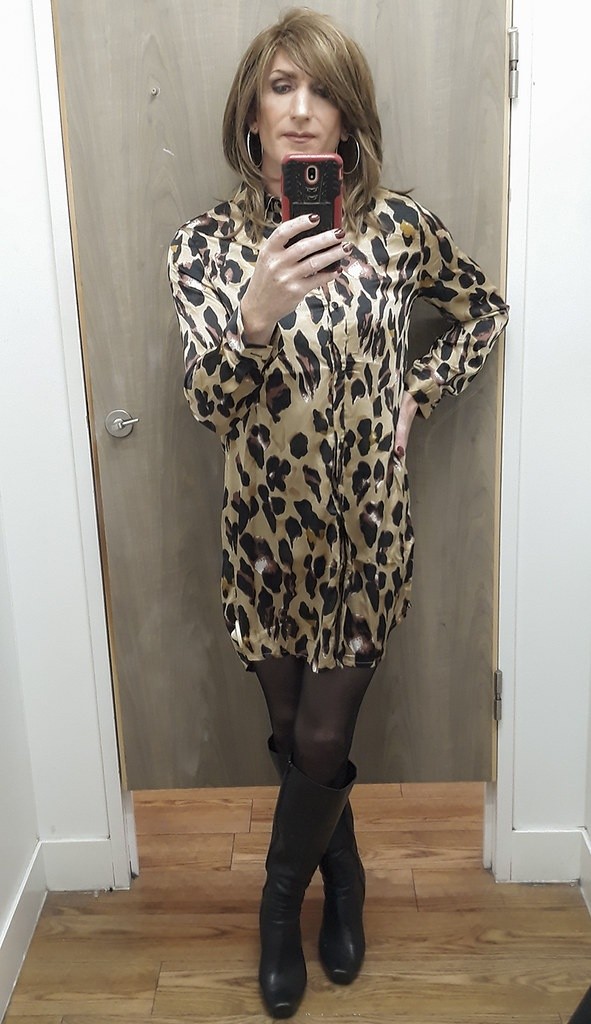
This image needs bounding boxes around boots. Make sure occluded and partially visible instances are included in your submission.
[259,752,357,1019]
[264,733,367,986]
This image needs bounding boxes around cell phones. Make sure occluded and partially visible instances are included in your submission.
[280,152,342,273]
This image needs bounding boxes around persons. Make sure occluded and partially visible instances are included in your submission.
[165,7,512,1017]
